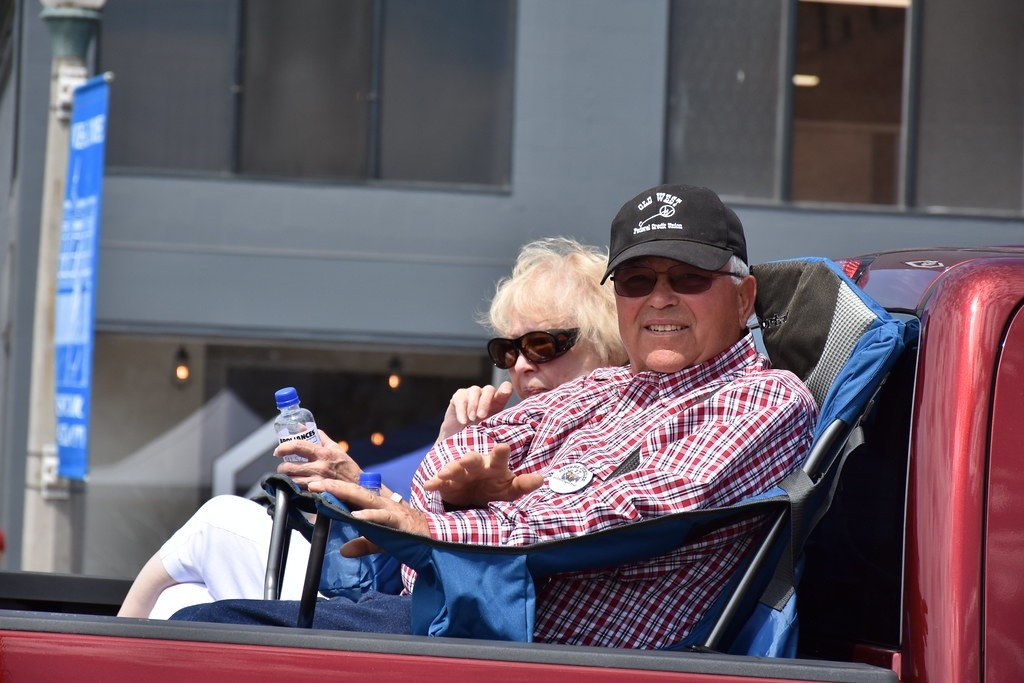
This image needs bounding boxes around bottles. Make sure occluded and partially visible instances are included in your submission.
[272,384,323,479]
[359,471,382,503]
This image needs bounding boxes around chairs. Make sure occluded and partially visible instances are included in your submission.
[259,254,907,654]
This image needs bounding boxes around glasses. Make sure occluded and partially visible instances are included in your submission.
[487,328,580,370]
[610,263,742,297]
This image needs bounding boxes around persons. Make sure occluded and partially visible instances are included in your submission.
[117,246,630,620]
[167,184,820,650]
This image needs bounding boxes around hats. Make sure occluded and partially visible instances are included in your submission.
[600,185,750,285]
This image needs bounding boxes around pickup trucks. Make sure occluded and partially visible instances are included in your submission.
[1,241,1024,682]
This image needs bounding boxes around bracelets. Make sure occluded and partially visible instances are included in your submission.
[390,492,402,503]
